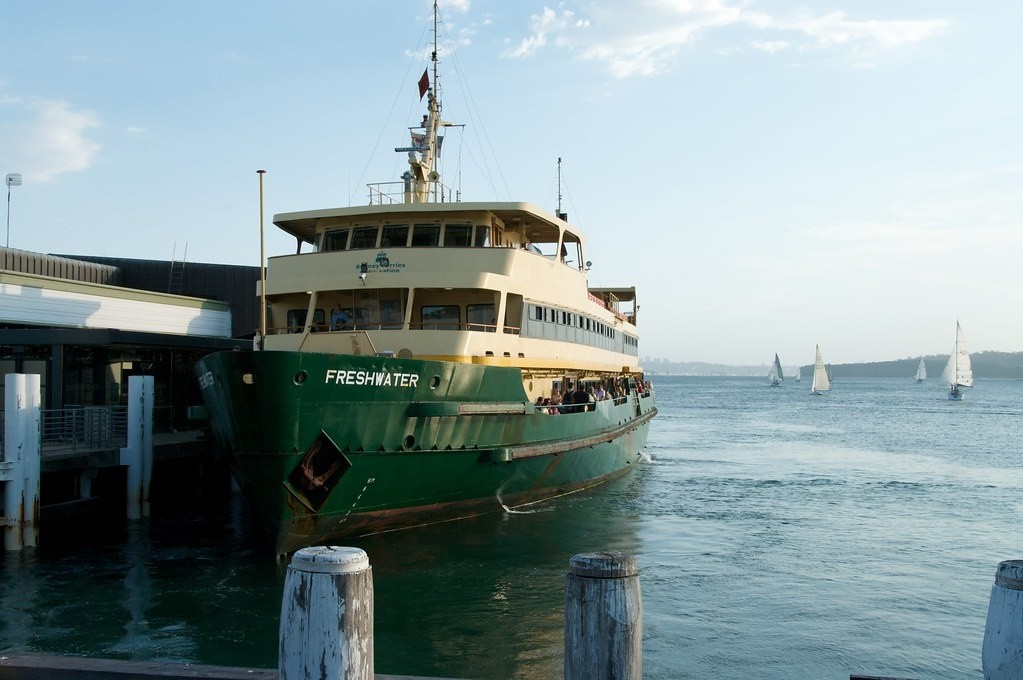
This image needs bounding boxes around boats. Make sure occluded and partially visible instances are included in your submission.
[237,0,659,556]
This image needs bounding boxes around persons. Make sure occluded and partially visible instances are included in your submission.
[330,303,353,331]
[534,379,654,415]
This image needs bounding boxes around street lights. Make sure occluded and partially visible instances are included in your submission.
[5,173,22,247]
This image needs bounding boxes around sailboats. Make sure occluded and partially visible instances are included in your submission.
[795,369,802,383]
[912,357,927,383]
[767,353,785,387]
[811,344,831,397]
[942,320,975,401]
